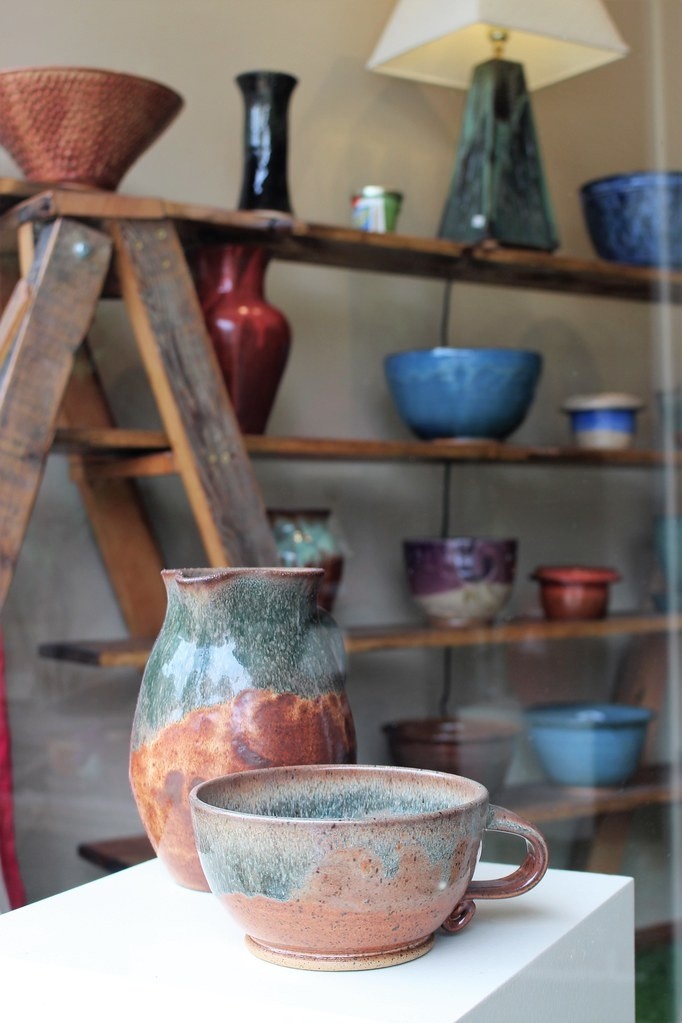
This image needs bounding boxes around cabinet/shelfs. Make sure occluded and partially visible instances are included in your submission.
[0,179,682,953]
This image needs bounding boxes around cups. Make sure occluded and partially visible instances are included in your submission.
[531,566,622,619]
[561,390,648,454]
[350,187,401,236]
[190,763,549,971]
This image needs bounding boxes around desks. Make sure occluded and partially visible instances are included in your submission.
[0,856,637,1023]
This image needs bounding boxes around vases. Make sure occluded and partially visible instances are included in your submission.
[234,71,297,218]
[194,239,292,437]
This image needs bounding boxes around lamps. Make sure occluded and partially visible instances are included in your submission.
[365,1,629,253]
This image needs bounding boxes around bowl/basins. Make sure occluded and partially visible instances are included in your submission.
[384,346,543,445]
[403,532,519,625]
[377,717,523,799]
[579,170,682,268]
[0,69,186,193]
[527,704,651,793]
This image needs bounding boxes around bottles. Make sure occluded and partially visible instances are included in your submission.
[235,69,298,219]
[130,566,357,892]
[265,504,345,611]
[189,237,291,437]
